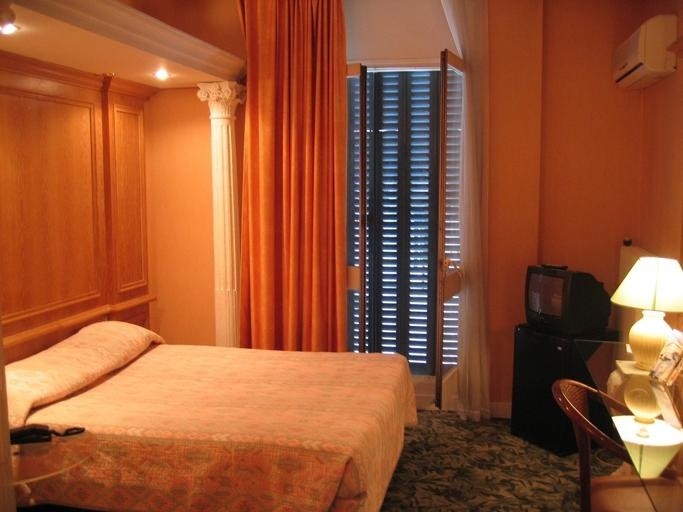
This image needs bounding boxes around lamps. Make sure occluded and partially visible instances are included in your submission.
[610,374,682,480]
[609,258,683,372]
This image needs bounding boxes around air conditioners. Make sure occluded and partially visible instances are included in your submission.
[611,13,678,91]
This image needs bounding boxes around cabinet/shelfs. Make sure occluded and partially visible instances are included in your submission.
[510,322,620,455]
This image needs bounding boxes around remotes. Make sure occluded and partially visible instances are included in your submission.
[541,264,568,271]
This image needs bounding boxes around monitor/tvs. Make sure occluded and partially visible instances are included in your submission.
[525,266,611,337]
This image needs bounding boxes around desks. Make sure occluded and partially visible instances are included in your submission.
[9,419,96,505]
[572,337,683,511]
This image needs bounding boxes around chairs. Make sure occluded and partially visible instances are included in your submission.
[549,377,683,509]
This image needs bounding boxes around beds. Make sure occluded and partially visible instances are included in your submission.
[3,320,411,512]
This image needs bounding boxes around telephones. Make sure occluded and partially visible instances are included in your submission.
[11,425,51,445]
